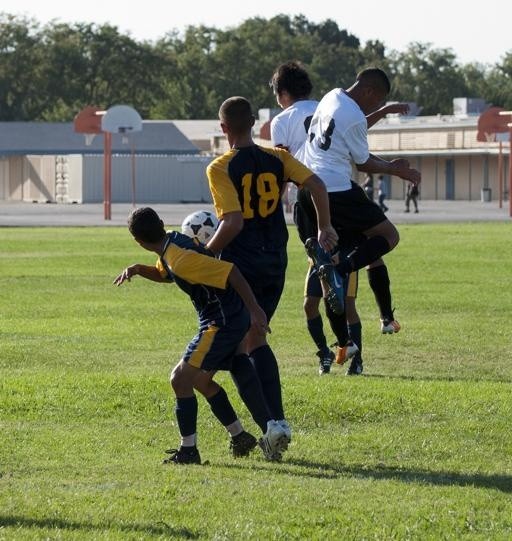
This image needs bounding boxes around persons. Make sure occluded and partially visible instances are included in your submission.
[295,64,422,369]
[404,180,420,214]
[257,57,368,378]
[374,173,388,215]
[197,97,340,464]
[111,207,275,465]
[362,172,374,200]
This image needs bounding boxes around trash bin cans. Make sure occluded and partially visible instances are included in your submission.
[480,187,492,202]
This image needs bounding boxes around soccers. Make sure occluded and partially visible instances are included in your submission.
[181,211,220,244]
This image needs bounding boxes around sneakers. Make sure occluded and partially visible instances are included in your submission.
[162,446,201,465]
[304,235,336,272]
[230,431,257,459]
[334,339,358,368]
[381,207,388,213]
[380,318,401,334]
[312,263,345,315]
[403,209,419,214]
[345,355,366,375]
[257,419,293,461]
[316,346,336,376]
